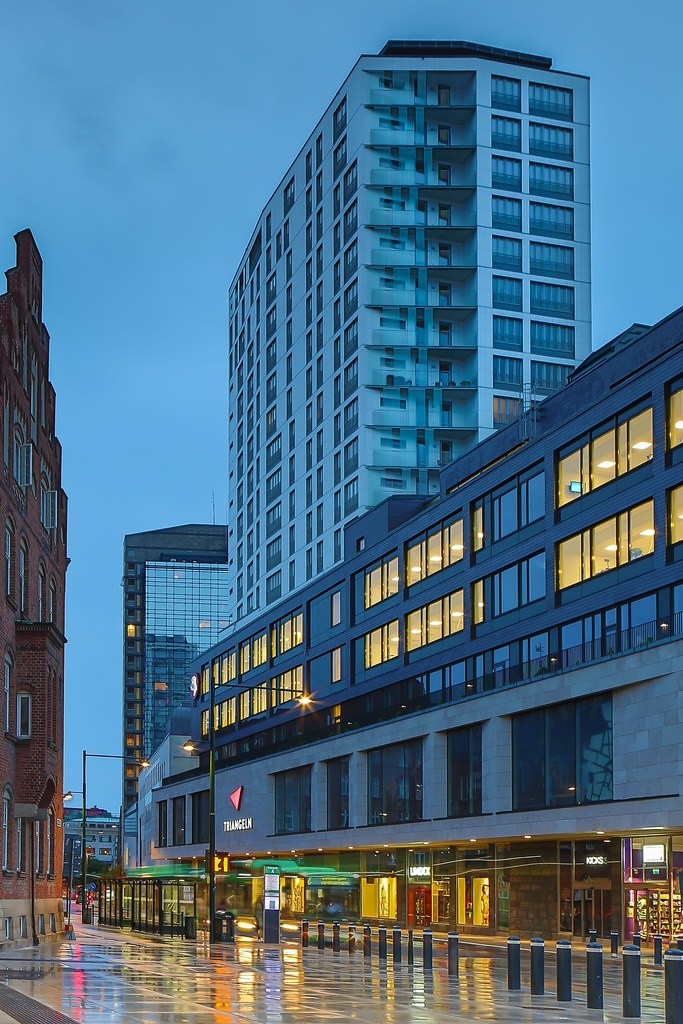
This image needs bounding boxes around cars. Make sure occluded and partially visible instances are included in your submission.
[76,892,93,905]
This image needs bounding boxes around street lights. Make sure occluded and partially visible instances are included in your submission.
[181,675,313,944]
[62,750,151,925]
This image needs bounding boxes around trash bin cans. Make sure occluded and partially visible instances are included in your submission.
[185,915,197,939]
[214,909,236,945]
[83,908,91,924]
